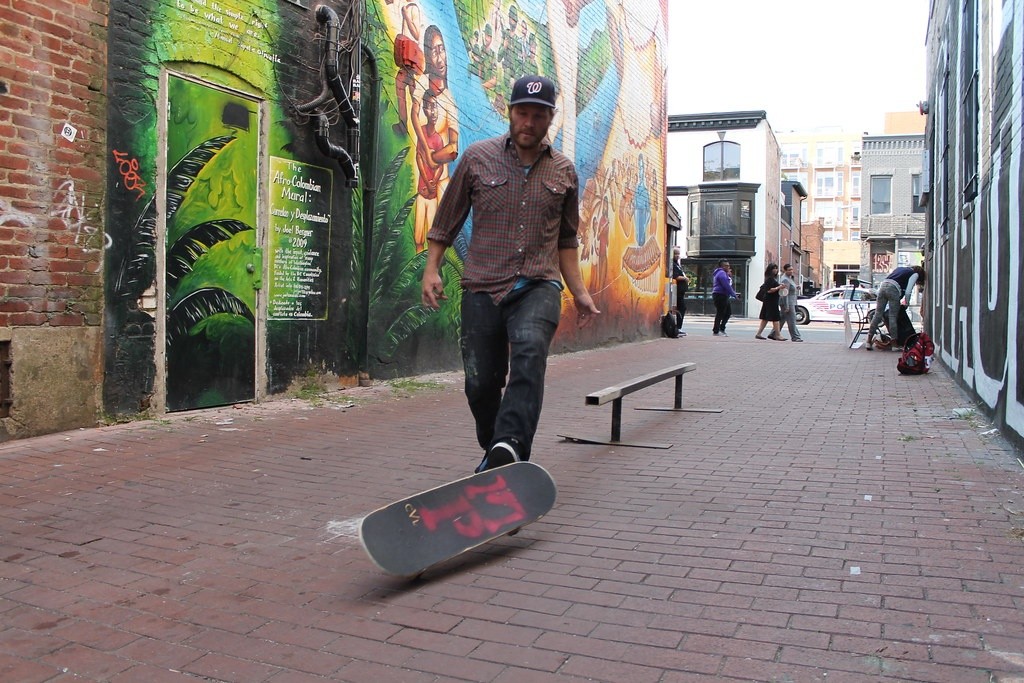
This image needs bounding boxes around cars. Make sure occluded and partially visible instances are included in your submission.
[795,285,913,328]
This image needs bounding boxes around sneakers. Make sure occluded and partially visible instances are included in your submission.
[773,335,785,341]
[866,342,873,351]
[791,337,804,342]
[712,330,729,337]
[677,329,688,336]
[892,346,900,352]
[767,334,774,339]
[755,335,767,339]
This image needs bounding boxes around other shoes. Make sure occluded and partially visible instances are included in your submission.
[392,124,408,137]
[473,449,489,475]
[485,437,522,470]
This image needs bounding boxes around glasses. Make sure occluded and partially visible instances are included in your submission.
[725,265,731,268]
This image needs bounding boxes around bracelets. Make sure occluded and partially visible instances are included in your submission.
[684,277,686,280]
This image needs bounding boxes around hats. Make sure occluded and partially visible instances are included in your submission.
[578,179,597,201]
[510,75,556,108]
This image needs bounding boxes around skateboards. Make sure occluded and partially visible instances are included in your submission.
[358,459,559,579]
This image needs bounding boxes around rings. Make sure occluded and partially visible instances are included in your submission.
[582,314,584,316]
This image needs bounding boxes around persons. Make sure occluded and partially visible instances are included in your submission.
[713,259,736,337]
[839,293,843,298]
[755,263,786,340]
[423,74,600,474]
[767,263,803,342]
[674,248,689,334]
[859,284,866,297]
[865,266,925,351]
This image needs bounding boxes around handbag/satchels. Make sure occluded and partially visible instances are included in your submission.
[755,285,774,306]
